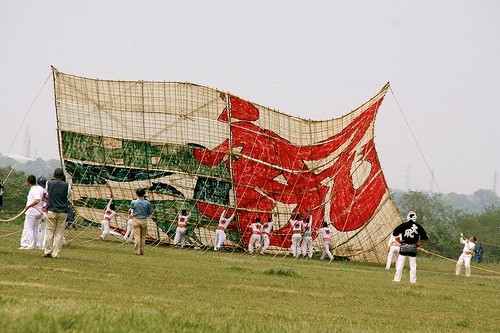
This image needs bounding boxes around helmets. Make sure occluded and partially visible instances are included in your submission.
[37,176,46,188]
[407,211,416,223]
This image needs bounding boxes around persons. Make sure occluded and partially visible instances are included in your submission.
[392,211,428,283]
[318,221,334,261]
[123,207,134,243]
[43,167,72,258]
[100,194,116,240]
[214,208,235,251]
[130,188,152,254]
[247,218,263,254]
[289,213,303,259]
[302,210,312,259]
[472,242,484,263]
[0,180,4,210]
[174,209,191,249]
[456,233,477,277]
[384,231,402,270]
[18,175,47,249]
[34,175,49,250]
[261,218,275,254]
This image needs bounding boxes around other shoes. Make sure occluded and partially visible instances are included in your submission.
[100,235,104,241]
[19,246,34,250]
